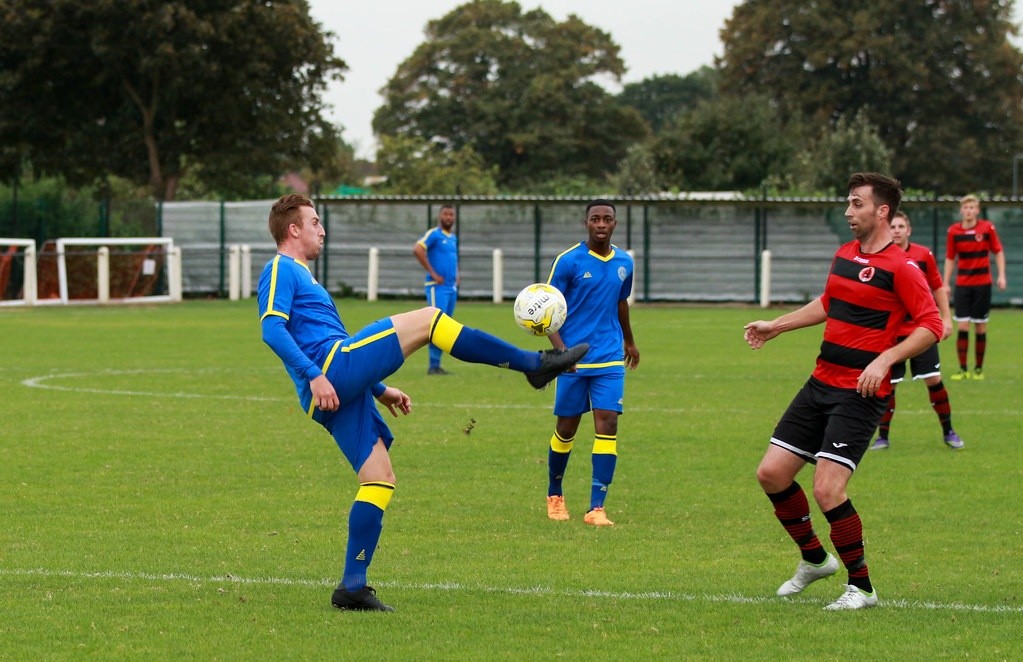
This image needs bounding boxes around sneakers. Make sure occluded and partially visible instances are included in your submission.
[952,371,971,381]
[821,585,878,612]
[426,368,452,376]
[775,553,839,597]
[584,508,615,527]
[548,494,571,524]
[528,343,590,390]
[972,369,984,380]
[944,431,963,449]
[331,583,395,615]
[871,439,888,451]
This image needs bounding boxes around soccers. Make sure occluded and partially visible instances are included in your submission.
[512,283,569,337]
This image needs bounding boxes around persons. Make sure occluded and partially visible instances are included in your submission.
[545,199,640,527]
[742,173,947,616]
[412,205,463,377]
[255,192,591,615]
[943,193,1008,384]
[869,210,967,454]
